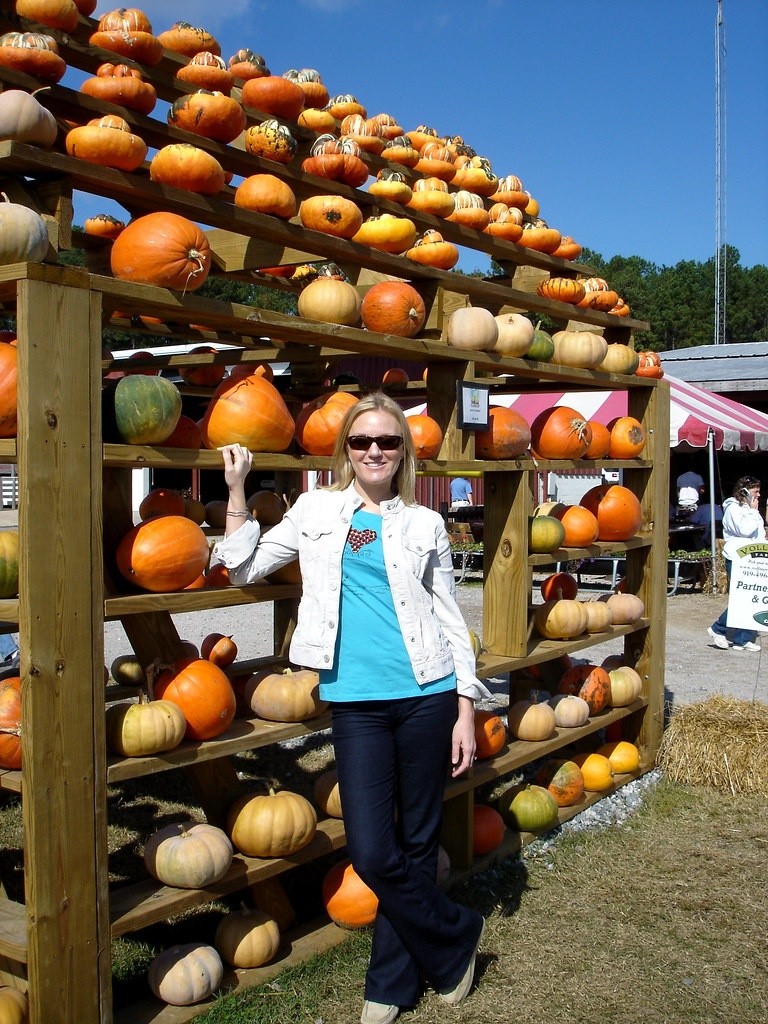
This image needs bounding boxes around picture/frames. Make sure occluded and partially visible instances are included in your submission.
[455,379,490,432]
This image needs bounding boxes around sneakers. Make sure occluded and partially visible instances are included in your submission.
[733,641,761,652]
[707,627,729,650]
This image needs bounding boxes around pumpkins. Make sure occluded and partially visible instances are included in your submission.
[0,0,664,766]
[143,655,642,1006]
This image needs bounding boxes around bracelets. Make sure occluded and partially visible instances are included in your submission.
[224,507,249,516]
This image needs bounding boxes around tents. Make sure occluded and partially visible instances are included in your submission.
[303,374,768,596]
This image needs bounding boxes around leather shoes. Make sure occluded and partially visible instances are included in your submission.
[360,999,399,1024]
[440,916,485,1004]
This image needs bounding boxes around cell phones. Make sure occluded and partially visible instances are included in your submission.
[742,488,752,501]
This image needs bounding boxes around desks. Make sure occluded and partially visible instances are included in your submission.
[669,524,708,550]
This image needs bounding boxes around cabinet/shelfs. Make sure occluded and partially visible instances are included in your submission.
[0,0,670,1024]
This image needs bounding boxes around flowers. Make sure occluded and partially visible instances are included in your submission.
[565,556,596,574]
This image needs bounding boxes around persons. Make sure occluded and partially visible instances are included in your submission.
[216,391,487,1024]
[450,477,473,507]
[707,475,768,652]
[0,634,20,668]
[670,462,723,545]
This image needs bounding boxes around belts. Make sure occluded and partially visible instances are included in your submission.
[453,499,467,502]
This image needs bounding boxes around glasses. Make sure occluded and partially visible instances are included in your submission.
[346,435,403,450]
